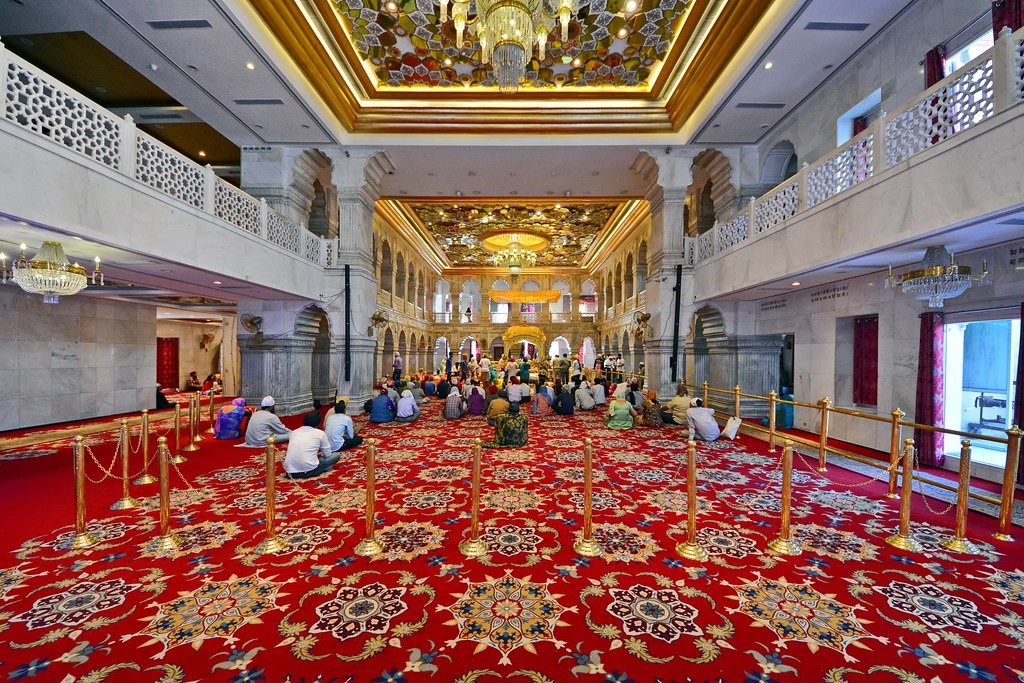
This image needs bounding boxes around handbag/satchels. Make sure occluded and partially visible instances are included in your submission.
[720,416,742,441]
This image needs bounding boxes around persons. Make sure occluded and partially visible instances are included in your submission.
[635,389,662,428]
[481,403,529,448]
[660,386,721,441]
[385,375,395,390]
[421,374,450,399]
[283,412,340,479]
[504,375,531,404]
[573,385,596,411]
[602,388,637,431]
[485,385,499,408]
[487,389,510,426]
[465,307,472,322]
[370,381,430,423]
[547,383,574,416]
[442,385,485,420]
[245,395,293,445]
[592,383,606,407]
[392,351,403,382]
[627,377,638,392]
[445,352,584,385]
[529,386,553,416]
[638,361,645,390]
[323,397,363,452]
[593,352,625,383]
[579,374,591,390]
[186,371,222,395]
[214,398,252,439]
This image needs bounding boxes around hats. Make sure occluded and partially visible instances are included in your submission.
[157,383,161,388]
[489,385,497,394]
[640,361,645,366]
[395,351,400,357]
[303,412,321,425]
[499,391,507,397]
[261,395,275,408]
[382,389,388,395]
[609,356,613,359]
[190,371,197,376]
[336,397,349,404]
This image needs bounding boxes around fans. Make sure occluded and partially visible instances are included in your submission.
[368,309,389,336]
[633,311,653,337]
[240,312,264,344]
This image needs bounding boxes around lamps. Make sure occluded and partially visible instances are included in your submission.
[438,0,572,93]
[0,241,105,304]
[888,246,988,308]
[488,233,537,285]
